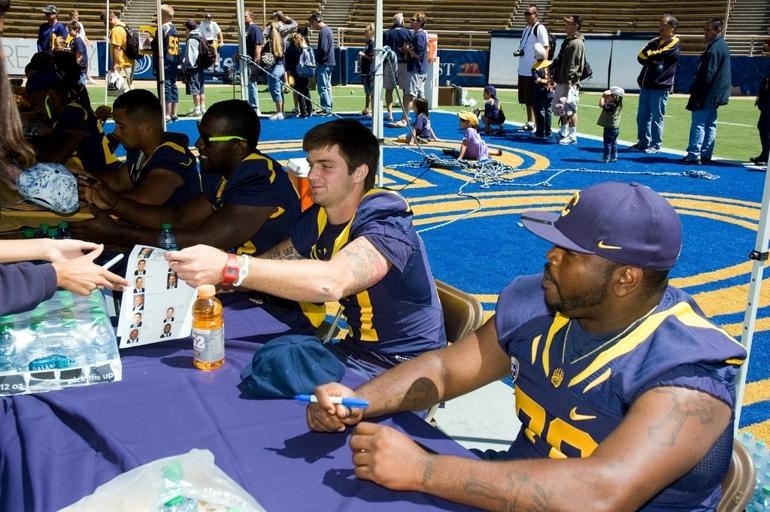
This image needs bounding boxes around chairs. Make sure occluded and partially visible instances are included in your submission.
[424,281,483,428]
[715,439,756,512]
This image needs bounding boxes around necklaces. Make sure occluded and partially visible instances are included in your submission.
[548,305,666,388]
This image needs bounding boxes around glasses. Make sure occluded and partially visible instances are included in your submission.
[524,11,536,16]
[410,17,417,22]
[196,120,248,146]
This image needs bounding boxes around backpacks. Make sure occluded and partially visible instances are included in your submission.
[126,30,144,59]
[295,47,317,78]
[198,36,216,69]
[546,33,557,60]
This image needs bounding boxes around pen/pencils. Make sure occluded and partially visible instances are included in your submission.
[72,173,97,184]
[102,254,124,270]
[294,394,370,410]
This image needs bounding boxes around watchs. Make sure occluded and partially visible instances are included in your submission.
[222,253,239,287]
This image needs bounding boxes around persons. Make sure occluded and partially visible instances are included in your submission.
[481,85,507,135]
[261,10,297,94]
[99,89,201,214]
[25,53,80,102]
[149,3,180,122]
[67,99,299,252]
[55,51,94,114]
[389,12,428,129]
[244,9,263,117]
[515,4,550,135]
[36,5,68,51]
[66,10,87,44]
[164,119,456,371]
[181,20,206,118]
[750,31,770,166]
[285,26,309,115]
[552,14,585,146]
[398,98,441,145]
[260,22,287,121]
[21,69,121,178]
[100,12,135,87]
[598,87,624,163]
[383,13,413,122]
[444,110,503,162]
[198,14,223,73]
[303,182,748,512]
[531,43,555,139]
[310,13,336,116]
[358,22,375,116]
[1,238,133,316]
[66,21,88,84]
[628,15,680,154]
[290,35,314,121]
[682,17,732,163]
[53,36,67,52]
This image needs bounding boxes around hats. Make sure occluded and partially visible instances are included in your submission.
[206,14,213,18]
[16,163,80,214]
[184,20,197,30]
[604,86,625,98]
[482,84,499,101]
[26,70,66,94]
[564,16,583,28]
[457,111,479,126]
[161,3,174,17]
[241,335,346,399]
[520,182,681,271]
[40,4,58,13]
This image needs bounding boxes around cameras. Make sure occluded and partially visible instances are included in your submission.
[512,48,525,59]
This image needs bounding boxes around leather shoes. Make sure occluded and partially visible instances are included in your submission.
[756,160,766,166]
[750,157,760,161]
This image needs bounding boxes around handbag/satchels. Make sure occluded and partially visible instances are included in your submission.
[424,31,435,63]
[581,56,593,81]
[262,41,275,72]
[554,88,579,116]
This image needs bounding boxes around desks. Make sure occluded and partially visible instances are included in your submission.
[1,290,481,512]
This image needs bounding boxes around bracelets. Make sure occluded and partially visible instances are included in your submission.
[233,254,249,287]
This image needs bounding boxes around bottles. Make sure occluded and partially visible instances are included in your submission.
[54,290,86,388]
[737,432,770,511]
[191,283,225,370]
[154,461,198,511]
[0,314,21,397]
[157,223,178,251]
[84,288,117,385]
[49,228,59,240]
[40,223,50,237]
[59,221,72,239]
[24,229,34,239]
[21,301,55,392]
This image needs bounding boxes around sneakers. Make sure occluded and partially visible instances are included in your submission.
[186,111,200,117]
[701,157,710,163]
[559,136,577,146]
[629,145,644,151]
[171,114,177,120]
[531,128,537,133]
[516,124,533,132]
[384,116,392,120]
[389,121,406,127]
[645,147,660,154]
[270,113,284,120]
[677,157,698,164]
[298,114,307,118]
[201,110,206,114]
[165,116,170,122]
[317,111,333,117]
[556,132,566,141]
